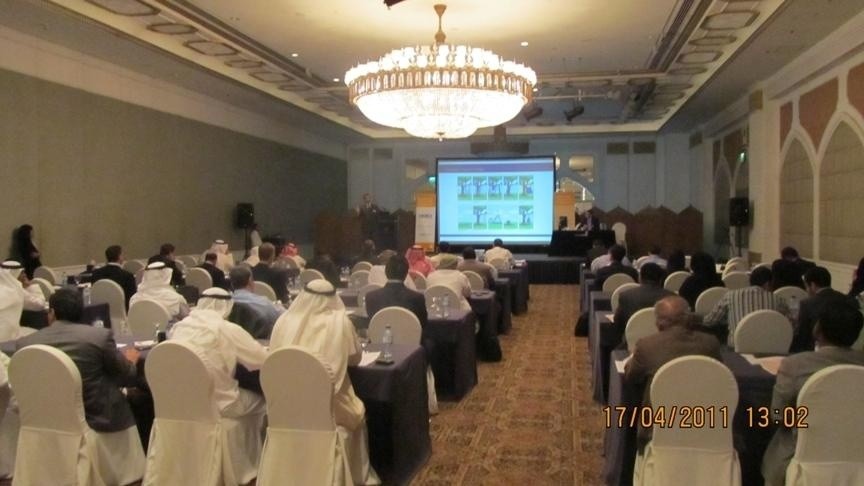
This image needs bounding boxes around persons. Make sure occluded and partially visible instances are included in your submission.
[632,244,669,276]
[679,252,725,312]
[249,223,263,248]
[789,267,860,354]
[761,301,862,483]
[768,246,817,295]
[3,239,513,368]
[167,287,270,419]
[13,224,42,281]
[353,193,380,252]
[580,210,600,231]
[623,295,724,457]
[703,266,791,353]
[15,288,151,433]
[457,177,532,229]
[270,279,366,430]
[847,258,862,313]
[613,263,677,351]
[590,240,632,278]
[594,245,638,291]
[660,252,690,288]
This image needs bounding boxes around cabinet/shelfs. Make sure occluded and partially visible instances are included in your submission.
[81,284,91,307]
[152,319,174,346]
[430,289,450,319]
[91,316,104,328]
[381,324,393,360]
[60,272,67,287]
[789,295,800,319]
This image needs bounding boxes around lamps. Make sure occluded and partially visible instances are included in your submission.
[611,221,628,256]
[341,3,538,143]
[521,87,609,123]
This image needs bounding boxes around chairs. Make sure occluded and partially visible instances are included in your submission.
[611,221,628,256]
[576,243,863,486]
[0,242,528,486]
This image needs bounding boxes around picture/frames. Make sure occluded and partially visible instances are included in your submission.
[547,229,615,258]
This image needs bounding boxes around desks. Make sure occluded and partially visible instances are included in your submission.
[547,229,615,258]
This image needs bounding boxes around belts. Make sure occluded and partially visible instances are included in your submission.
[357,328,370,362]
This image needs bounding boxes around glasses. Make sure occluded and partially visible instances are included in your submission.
[341,3,538,143]
[521,87,609,123]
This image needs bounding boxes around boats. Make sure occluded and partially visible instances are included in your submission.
[729,197,748,226]
[235,202,254,228]
